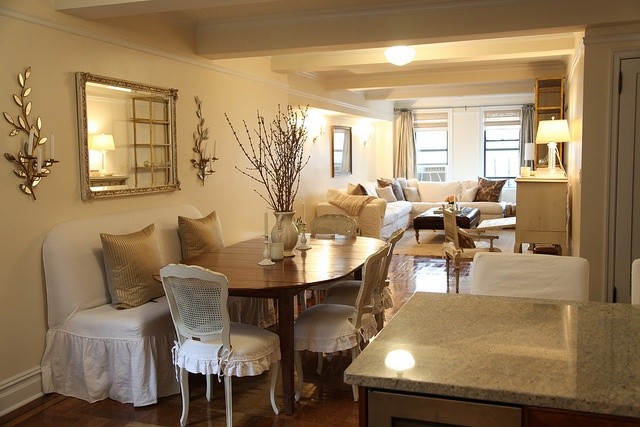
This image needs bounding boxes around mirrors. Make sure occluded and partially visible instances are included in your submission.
[331,125,353,178]
[76,71,182,201]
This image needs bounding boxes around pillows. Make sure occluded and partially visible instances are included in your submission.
[396,179,406,188]
[178,210,224,260]
[376,185,397,203]
[347,183,368,196]
[403,186,421,201]
[474,177,506,202]
[100,223,165,309]
[376,178,404,201]
[462,186,480,201]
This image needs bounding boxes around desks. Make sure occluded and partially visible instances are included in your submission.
[343,290,640,427]
[178,232,387,416]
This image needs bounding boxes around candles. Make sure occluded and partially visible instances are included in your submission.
[212,140,218,158]
[36,146,43,174]
[209,153,213,172]
[48,131,56,161]
[301,202,306,224]
[263,212,269,241]
[203,143,207,159]
[28,126,35,157]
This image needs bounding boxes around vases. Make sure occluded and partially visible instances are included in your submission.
[270,211,298,258]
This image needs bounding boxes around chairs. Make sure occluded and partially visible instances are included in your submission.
[470,252,590,303]
[442,204,502,294]
[322,228,405,331]
[631,259,640,305]
[300,213,358,311]
[159,263,281,427]
[294,242,391,402]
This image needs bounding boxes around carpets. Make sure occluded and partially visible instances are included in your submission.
[389,225,535,259]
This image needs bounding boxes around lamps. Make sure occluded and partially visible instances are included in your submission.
[534,116,570,178]
[92,133,115,176]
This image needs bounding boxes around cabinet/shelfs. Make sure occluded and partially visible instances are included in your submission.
[534,77,564,168]
[133,95,171,187]
[513,171,570,257]
[90,175,130,187]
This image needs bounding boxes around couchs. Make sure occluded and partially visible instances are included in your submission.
[317,178,505,241]
[39,203,278,408]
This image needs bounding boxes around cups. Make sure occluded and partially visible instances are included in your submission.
[269,242,285,262]
[521,167,531,177]
